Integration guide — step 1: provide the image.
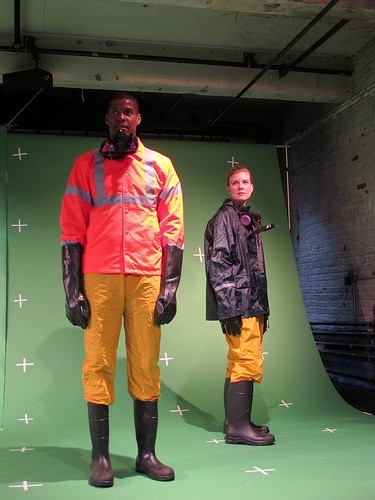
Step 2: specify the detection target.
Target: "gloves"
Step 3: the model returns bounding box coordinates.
[220,315,242,337]
[61,241,90,329]
[154,245,183,325]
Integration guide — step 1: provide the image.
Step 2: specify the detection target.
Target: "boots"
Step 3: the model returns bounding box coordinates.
[224,379,276,445]
[88,402,114,487]
[223,376,270,434]
[134,399,175,482]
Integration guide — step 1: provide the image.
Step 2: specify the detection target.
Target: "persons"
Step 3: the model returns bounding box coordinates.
[202,166,277,444]
[60,94,184,490]
